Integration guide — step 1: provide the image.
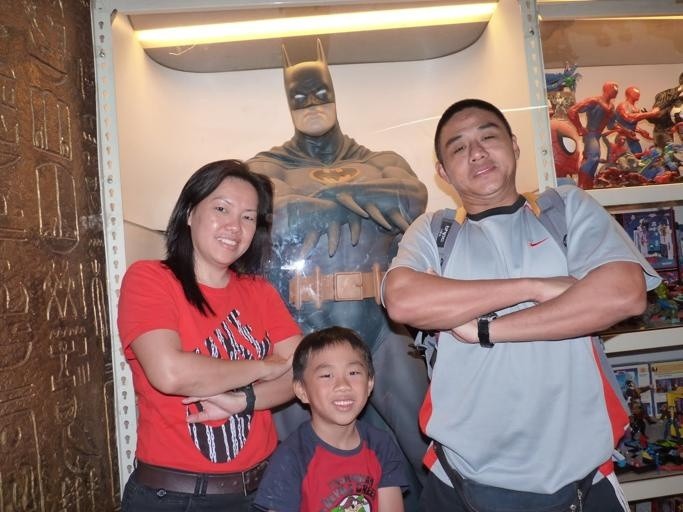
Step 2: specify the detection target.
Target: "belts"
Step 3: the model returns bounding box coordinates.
[135,460,269,497]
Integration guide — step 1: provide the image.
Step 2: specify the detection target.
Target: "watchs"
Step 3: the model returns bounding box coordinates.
[474,311,497,349]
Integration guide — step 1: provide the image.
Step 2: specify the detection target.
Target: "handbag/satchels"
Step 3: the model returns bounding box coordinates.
[453,473,590,511]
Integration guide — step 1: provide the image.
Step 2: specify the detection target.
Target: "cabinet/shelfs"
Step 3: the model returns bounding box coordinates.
[540,0,683,512]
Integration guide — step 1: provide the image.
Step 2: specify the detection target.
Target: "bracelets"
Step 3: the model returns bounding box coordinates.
[236,386,256,417]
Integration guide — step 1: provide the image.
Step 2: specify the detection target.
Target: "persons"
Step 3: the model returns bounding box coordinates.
[378,96,664,512]
[252,324,412,512]
[116,156,309,512]
